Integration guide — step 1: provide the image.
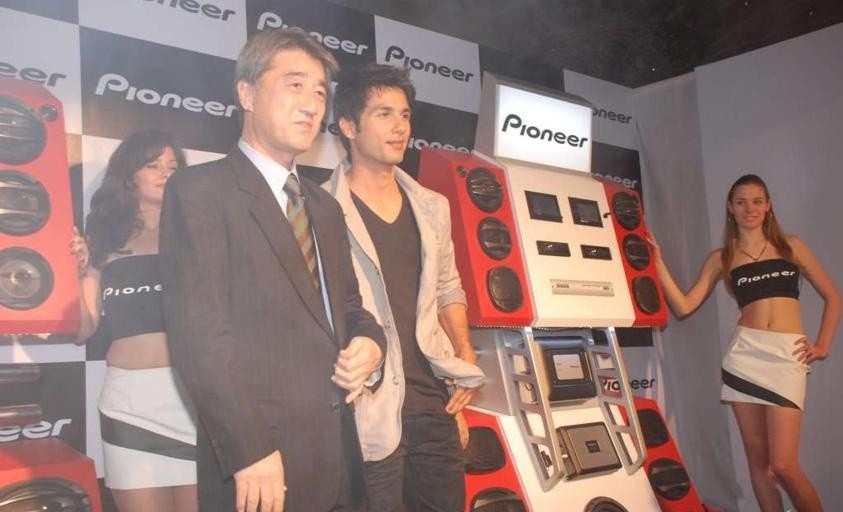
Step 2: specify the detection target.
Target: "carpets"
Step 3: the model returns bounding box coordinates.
[282,174,326,313]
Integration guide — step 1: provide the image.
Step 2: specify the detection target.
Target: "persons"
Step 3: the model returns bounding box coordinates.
[64,125,204,512]
[154,24,393,512]
[297,59,491,511]
[636,169,841,511]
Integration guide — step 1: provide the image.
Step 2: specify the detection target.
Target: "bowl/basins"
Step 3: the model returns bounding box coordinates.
[738,238,771,261]
